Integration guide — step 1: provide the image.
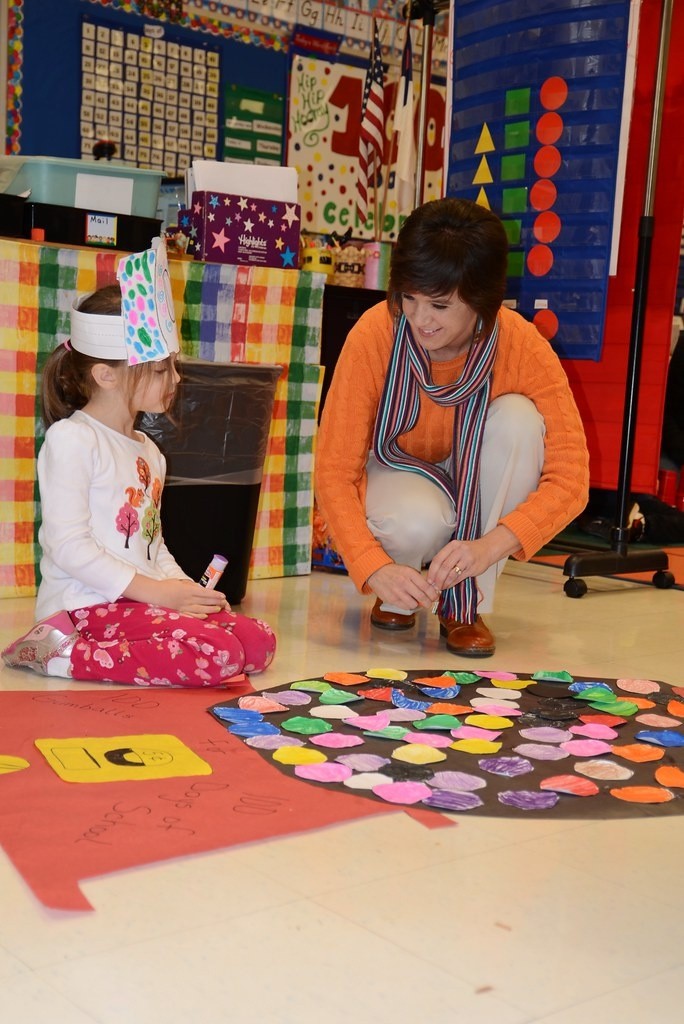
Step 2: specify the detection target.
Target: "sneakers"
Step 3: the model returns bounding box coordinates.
[0,610,81,678]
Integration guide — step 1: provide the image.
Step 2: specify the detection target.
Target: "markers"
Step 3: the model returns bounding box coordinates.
[199,555,228,590]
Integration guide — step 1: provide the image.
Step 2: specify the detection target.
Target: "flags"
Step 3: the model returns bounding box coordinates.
[359,23,417,219]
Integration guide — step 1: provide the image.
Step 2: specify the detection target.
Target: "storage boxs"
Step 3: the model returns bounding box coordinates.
[178,191,301,269]
[0,153,170,253]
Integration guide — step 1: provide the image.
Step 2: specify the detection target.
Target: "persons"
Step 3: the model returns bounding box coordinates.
[0,285,277,689]
[313,200,589,656]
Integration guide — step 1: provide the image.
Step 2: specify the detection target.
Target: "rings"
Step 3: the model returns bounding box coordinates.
[453,565,462,575]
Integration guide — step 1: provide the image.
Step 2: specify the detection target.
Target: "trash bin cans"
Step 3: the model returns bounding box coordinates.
[134,359,284,606]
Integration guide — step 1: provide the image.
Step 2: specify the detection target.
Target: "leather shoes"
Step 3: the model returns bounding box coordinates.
[371,595,416,630]
[438,612,495,656]
